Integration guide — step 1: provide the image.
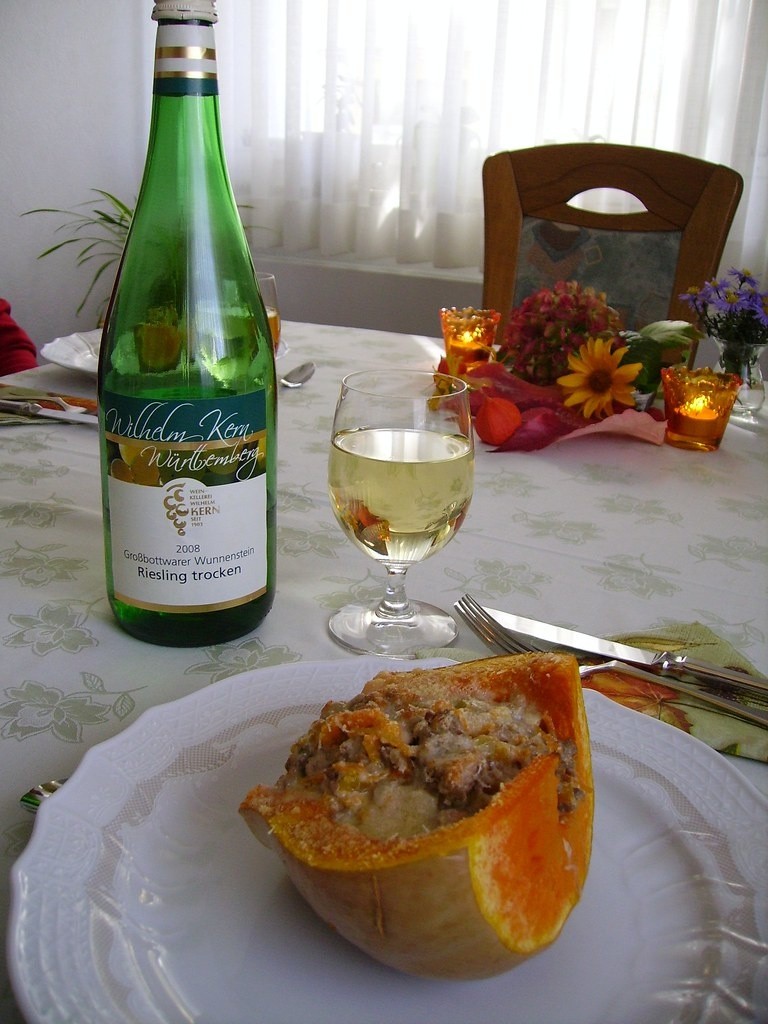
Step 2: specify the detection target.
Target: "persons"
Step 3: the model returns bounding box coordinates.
[1,297,40,378]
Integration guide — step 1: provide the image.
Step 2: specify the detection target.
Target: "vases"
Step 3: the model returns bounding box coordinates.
[712,336,766,418]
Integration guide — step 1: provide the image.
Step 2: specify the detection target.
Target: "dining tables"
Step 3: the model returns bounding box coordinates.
[1,318,767,1024]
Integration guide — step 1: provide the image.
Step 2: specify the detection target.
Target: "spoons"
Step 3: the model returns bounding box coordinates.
[281,360,317,387]
[0,393,97,415]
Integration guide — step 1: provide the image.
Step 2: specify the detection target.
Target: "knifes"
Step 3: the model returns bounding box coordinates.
[0,400,98,422]
[457,602,767,701]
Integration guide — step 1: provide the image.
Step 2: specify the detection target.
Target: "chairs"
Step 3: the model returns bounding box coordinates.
[481,142,745,371]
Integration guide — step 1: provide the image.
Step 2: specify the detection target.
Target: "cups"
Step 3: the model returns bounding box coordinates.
[661,366,742,451]
[253,270,281,358]
[441,307,502,382]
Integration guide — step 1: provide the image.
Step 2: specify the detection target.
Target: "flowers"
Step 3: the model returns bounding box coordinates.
[430,280,705,452]
[680,267,768,401]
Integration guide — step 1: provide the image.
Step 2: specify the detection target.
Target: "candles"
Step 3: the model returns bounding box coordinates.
[449,331,484,362]
[672,395,719,437]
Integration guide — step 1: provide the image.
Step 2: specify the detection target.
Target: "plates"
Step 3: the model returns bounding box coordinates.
[41,328,290,380]
[1,653,767,1024]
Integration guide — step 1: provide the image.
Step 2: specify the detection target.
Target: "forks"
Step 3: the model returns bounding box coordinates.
[452,594,768,728]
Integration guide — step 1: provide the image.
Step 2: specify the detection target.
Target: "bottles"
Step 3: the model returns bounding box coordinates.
[94,1,281,649]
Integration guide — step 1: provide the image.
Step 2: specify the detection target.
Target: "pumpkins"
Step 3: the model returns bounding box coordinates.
[244,630,596,981]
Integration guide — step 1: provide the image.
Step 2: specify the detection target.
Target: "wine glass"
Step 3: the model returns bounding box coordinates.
[328,370,475,658]
